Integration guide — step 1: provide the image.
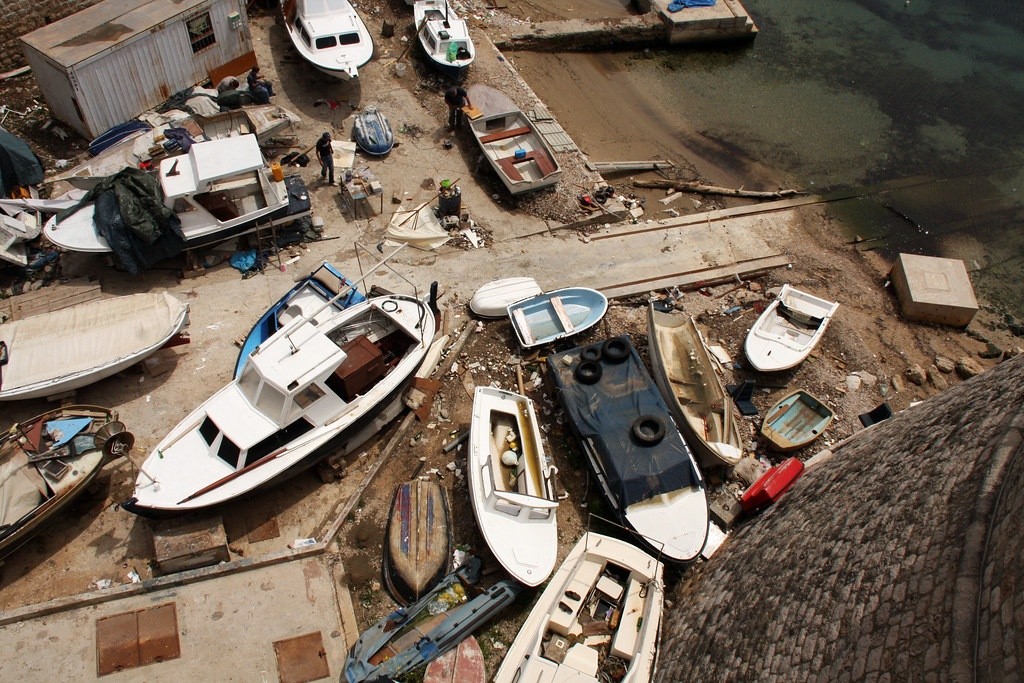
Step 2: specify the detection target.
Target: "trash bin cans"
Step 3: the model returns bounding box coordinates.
[438,185,461,217]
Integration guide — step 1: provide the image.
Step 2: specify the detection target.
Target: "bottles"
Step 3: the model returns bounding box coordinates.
[526,365,540,372]
[414,431,422,441]
[449,428,460,435]
[436,471,444,480]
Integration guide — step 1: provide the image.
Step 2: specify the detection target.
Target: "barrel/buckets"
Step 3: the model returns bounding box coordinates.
[438,188,461,219]
[271,161,284,182]
[396,63,406,77]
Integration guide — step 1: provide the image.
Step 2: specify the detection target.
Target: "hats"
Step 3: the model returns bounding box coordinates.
[323,133,330,140]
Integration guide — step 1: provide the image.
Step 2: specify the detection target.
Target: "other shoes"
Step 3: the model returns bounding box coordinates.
[268,92,277,96]
[323,173,327,180]
[329,182,338,186]
[447,125,454,132]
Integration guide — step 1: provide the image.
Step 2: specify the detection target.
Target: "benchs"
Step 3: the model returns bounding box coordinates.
[513,307,534,344]
[479,123,530,146]
[550,296,574,333]
[493,147,553,181]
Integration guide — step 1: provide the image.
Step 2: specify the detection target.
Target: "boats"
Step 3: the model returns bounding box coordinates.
[544,335,709,578]
[0,402,118,566]
[36,101,303,190]
[413,0,475,81]
[234,258,365,384]
[121,238,441,519]
[384,476,452,606]
[279,0,374,82]
[760,388,835,454]
[469,276,542,318]
[423,635,486,683]
[465,84,562,196]
[0,292,190,400]
[507,286,608,349]
[647,302,743,472]
[43,132,312,254]
[744,281,841,378]
[490,512,665,683]
[353,105,394,155]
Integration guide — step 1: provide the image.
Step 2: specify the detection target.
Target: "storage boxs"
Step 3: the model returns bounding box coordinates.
[152,515,231,574]
[889,253,979,329]
[329,335,385,398]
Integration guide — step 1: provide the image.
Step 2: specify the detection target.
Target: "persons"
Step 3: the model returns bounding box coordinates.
[445,86,473,133]
[247,68,276,104]
[217,77,240,95]
[316,132,339,186]
